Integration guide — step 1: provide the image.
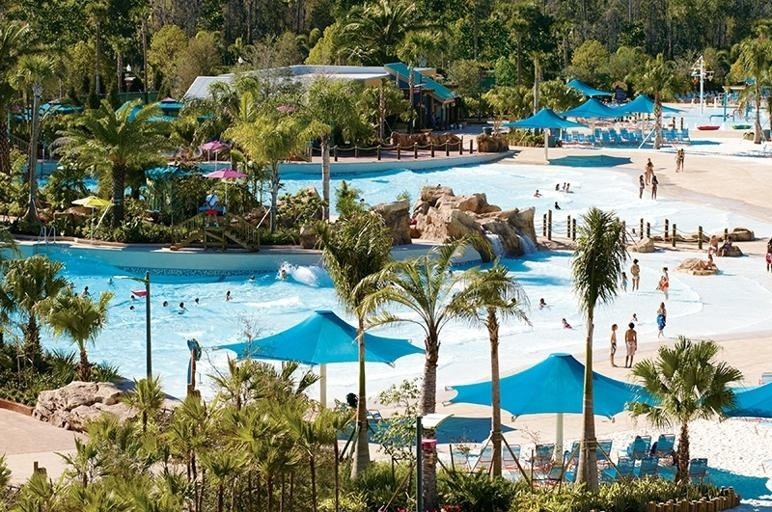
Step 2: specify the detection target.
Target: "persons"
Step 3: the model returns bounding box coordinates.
[562,318,571,328]
[431,112,437,126]
[533,182,572,209]
[638,158,658,199]
[690,235,732,270]
[631,259,640,292]
[205,189,220,229]
[657,267,669,291]
[620,272,628,292]
[73,267,287,315]
[632,313,637,321]
[539,299,546,309]
[657,302,667,338]
[766,238,772,272]
[674,149,684,173]
[610,323,637,366]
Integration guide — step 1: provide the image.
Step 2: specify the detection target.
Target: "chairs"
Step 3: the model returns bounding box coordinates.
[554,127,692,147]
[453,434,708,485]
[333,398,384,423]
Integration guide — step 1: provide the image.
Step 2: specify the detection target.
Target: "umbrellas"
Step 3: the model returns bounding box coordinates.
[70,196,114,240]
[556,98,637,146]
[441,353,663,463]
[215,309,426,409]
[565,79,613,97]
[198,139,233,171]
[202,167,249,206]
[618,94,689,143]
[502,107,589,161]
[698,379,772,419]
